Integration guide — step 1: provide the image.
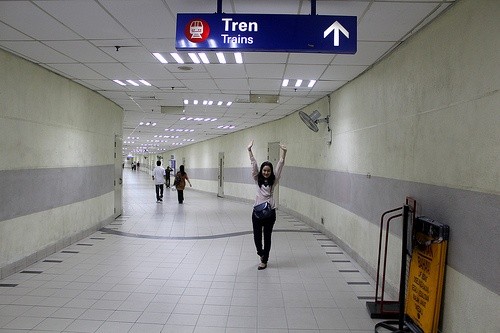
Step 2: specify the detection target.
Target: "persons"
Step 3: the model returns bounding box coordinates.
[247,137,288,271]
[131,160,140,172]
[151,160,167,204]
[164,165,173,188]
[173,165,193,205]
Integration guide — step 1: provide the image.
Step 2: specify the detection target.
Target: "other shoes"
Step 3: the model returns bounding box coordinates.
[257,250,267,270]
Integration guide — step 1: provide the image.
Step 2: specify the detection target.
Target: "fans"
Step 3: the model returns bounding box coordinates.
[156,155,162,161]
[298,109,330,134]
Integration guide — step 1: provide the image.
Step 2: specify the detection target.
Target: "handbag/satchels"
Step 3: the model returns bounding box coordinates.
[254,201,272,220]
